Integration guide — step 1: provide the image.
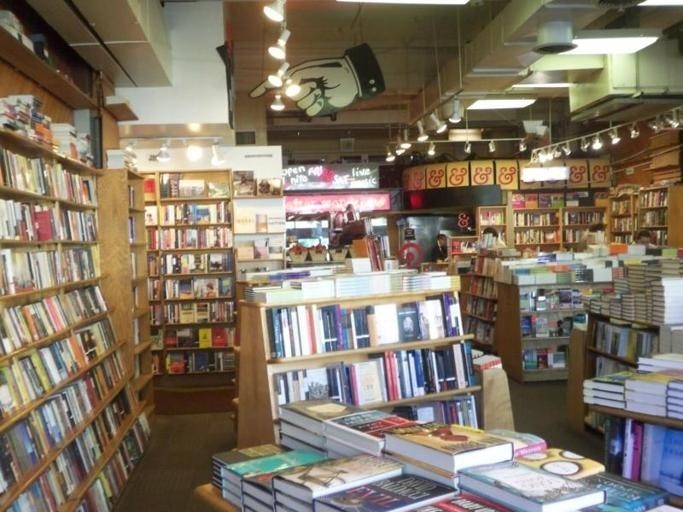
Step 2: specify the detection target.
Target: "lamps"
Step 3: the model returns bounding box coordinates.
[530,106,683,167]
[559,26,664,57]
[467,95,538,110]
[263,1,301,113]
[385,4,465,162]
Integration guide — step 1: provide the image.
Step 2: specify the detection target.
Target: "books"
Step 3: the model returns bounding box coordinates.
[143,174,236,375]
[212,257,670,512]
[0,94,151,512]
[584,353,683,498]
[453,189,683,377]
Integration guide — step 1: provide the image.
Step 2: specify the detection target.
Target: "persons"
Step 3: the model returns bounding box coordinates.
[432,234,448,263]
